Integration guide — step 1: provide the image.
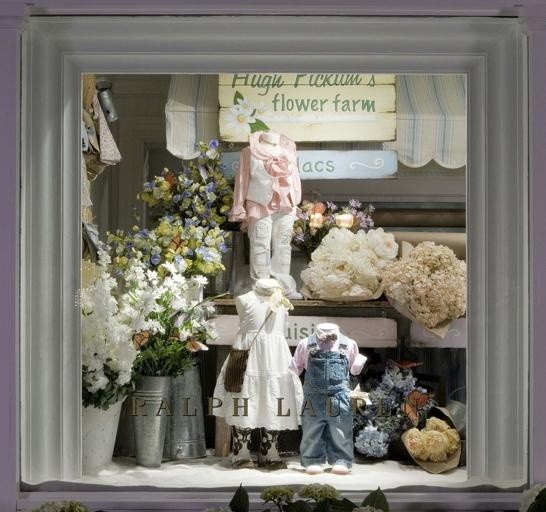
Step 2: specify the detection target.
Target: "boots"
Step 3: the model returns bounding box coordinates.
[231,426,254,469]
[257,427,288,470]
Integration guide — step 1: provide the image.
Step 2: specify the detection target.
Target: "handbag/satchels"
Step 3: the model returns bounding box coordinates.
[225,348,249,393]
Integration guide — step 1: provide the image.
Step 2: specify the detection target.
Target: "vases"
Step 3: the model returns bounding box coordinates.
[165,364,206,459]
[131,375,170,466]
[82,398,128,473]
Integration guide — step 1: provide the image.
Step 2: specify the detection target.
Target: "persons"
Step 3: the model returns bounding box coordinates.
[212,279,304,469]
[228,128,303,302]
[286,322,369,475]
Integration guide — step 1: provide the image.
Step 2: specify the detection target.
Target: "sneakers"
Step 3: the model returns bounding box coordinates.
[330,465,348,475]
[284,291,304,300]
[306,464,323,474]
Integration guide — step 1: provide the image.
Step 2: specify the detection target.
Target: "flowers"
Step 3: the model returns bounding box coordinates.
[71,138,238,412]
[399,417,463,475]
[221,482,389,512]
[224,91,272,131]
[350,363,438,460]
[289,196,467,340]
